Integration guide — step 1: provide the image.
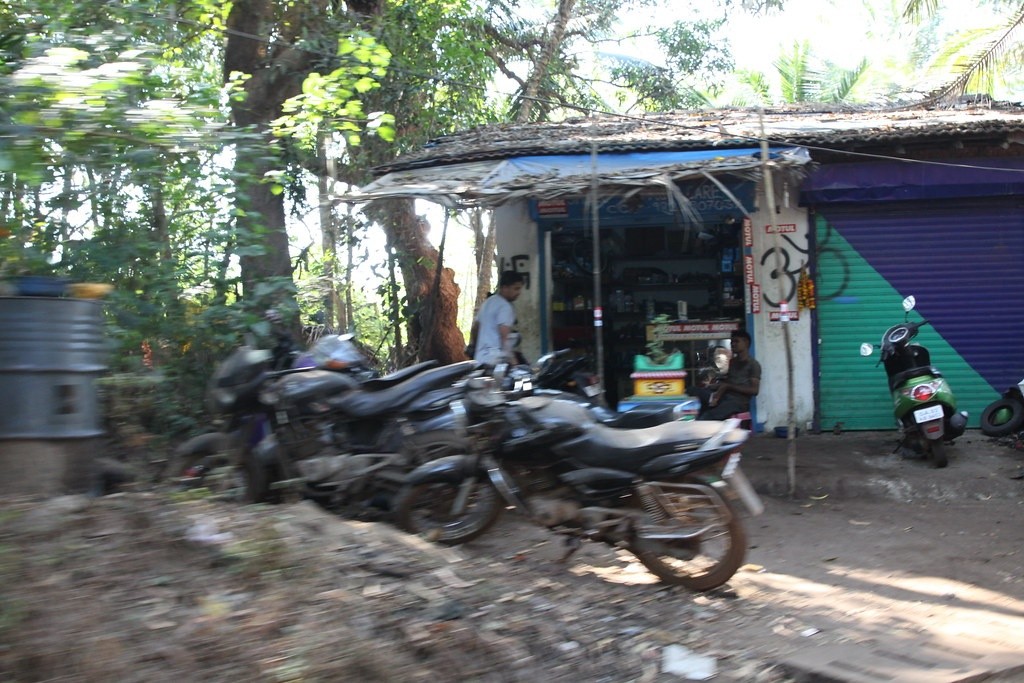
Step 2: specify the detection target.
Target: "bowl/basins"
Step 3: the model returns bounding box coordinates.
[0,272,69,296]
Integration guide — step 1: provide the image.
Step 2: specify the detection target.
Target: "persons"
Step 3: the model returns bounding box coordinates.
[472,272,528,367]
[687,330,761,421]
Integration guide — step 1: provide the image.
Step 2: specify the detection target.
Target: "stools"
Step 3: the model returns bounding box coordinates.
[730,411,754,432]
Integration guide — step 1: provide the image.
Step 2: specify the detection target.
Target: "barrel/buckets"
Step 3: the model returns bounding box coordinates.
[0,296,106,499]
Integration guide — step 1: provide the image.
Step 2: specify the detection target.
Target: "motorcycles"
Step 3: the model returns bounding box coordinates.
[158,308,765,591]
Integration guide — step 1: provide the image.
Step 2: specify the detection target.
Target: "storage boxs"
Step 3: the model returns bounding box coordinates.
[620,372,700,419]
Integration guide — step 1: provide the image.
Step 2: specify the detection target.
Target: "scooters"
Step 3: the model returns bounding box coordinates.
[859,295,970,470]
